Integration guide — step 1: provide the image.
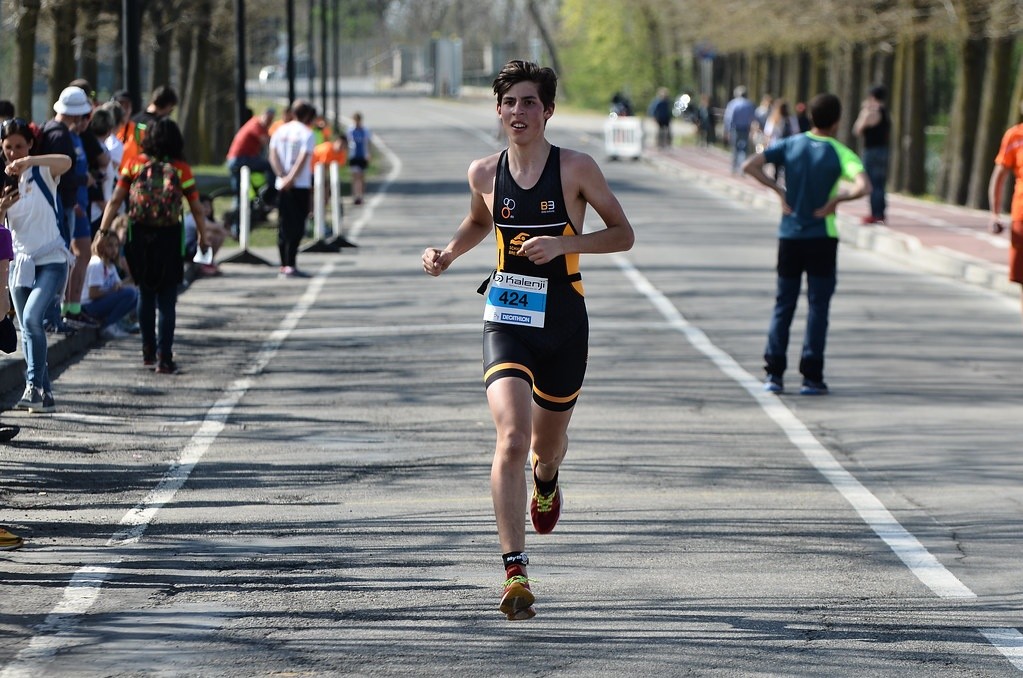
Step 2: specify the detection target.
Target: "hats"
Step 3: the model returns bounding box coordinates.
[53,87,92,115]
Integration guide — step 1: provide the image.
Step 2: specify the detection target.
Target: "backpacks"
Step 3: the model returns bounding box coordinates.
[129,153,181,225]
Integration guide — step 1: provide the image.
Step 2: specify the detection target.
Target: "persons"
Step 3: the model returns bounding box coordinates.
[68,79,92,98]
[269,102,317,279]
[266,105,294,137]
[1,116,72,413]
[421,59,635,621]
[102,101,126,179]
[38,87,93,335]
[721,85,754,178]
[110,214,139,333]
[226,105,276,240]
[346,112,372,204]
[988,94,1023,285]
[608,85,715,163]
[763,100,800,184]
[91,108,115,238]
[92,120,213,374]
[851,88,890,224]
[111,90,145,174]
[308,135,348,220]
[743,93,872,398]
[184,193,225,277]
[134,85,185,160]
[80,231,140,339]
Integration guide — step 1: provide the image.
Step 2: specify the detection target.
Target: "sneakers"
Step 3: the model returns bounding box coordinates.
[157,362,177,373]
[500,565,537,620]
[280,266,312,278]
[531,452,562,534]
[18,388,43,407]
[144,359,159,369]
[108,323,131,338]
[28,392,56,412]
[62,311,100,329]
[801,385,829,394]
[765,382,783,392]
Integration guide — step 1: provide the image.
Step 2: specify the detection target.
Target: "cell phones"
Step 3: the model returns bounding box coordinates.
[6,174,19,198]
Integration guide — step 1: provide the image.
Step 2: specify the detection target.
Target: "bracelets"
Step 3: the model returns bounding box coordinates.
[98,227,108,238]
